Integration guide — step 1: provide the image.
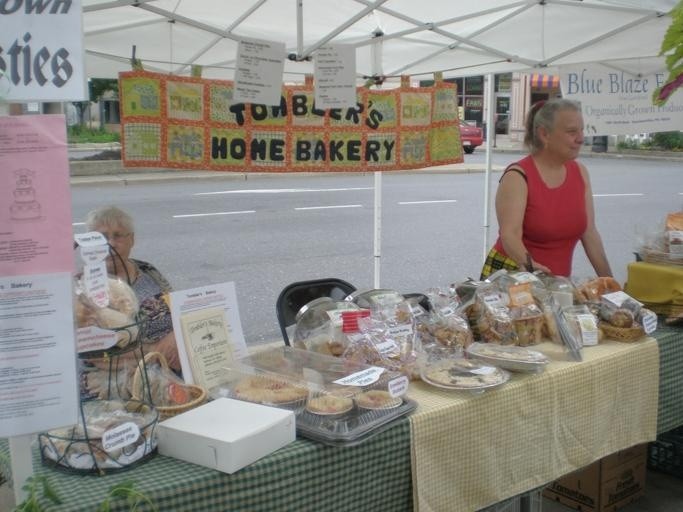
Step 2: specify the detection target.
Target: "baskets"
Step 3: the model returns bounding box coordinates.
[95,350,209,423]
[597,317,646,343]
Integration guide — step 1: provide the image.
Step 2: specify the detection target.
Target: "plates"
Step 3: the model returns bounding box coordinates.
[465,343,546,374]
[421,365,511,394]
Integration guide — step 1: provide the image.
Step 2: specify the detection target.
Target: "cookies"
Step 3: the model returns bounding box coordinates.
[308,275,640,380]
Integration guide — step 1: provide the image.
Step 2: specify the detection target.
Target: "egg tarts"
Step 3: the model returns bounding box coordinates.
[358,389,400,415]
[235,374,280,400]
[307,395,355,420]
[265,381,310,406]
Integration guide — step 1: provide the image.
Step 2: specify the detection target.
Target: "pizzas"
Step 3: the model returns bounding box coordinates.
[426,342,542,387]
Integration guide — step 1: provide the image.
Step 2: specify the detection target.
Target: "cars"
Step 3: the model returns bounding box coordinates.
[459,117,484,153]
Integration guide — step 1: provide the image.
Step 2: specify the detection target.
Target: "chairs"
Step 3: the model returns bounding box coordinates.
[274,279,361,347]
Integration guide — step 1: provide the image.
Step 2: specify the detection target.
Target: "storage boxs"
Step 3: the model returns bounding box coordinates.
[538,441,647,512]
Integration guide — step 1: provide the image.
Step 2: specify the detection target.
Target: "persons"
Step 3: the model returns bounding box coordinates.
[71,204,181,401]
[482,95,612,279]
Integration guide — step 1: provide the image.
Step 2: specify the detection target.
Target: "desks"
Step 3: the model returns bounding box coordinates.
[623,253,683,323]
[409,323,658,512]
[30,376,409,511]
[648,321,682,436]
